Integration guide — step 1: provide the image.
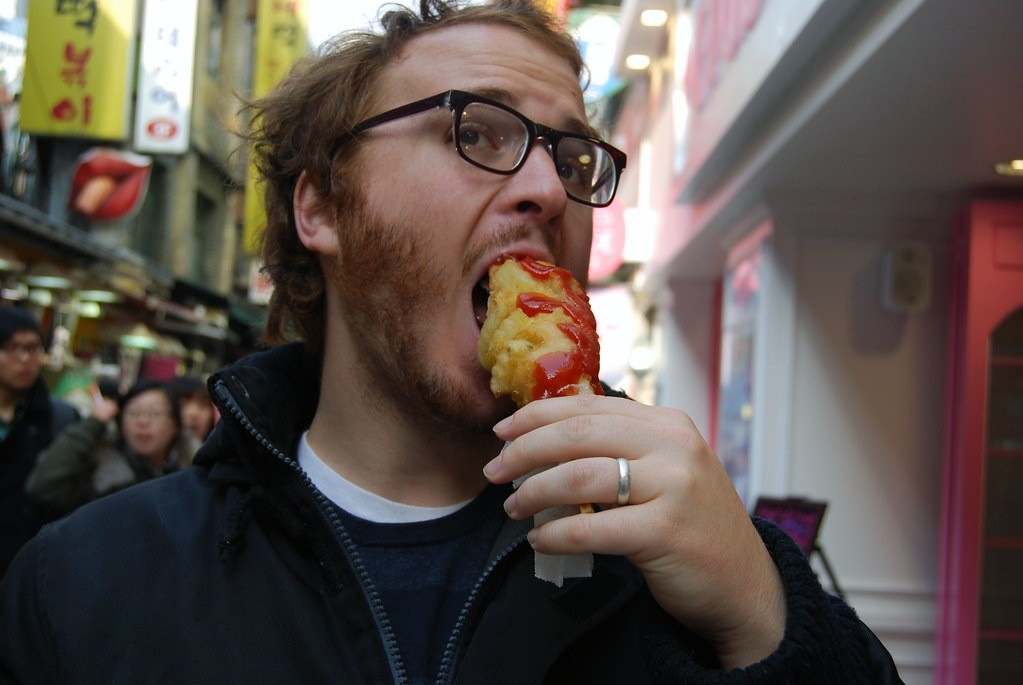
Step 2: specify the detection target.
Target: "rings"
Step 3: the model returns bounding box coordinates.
[618,457,631,505]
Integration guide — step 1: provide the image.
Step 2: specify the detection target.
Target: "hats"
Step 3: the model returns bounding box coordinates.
[0,305,41,355]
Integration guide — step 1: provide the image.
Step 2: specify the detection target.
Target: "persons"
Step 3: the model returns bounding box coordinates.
[0,0,907,683]
[1,302,222,549]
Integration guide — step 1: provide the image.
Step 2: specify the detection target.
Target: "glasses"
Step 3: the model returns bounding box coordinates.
[322,90,629,207]
[0,345,45,362]
